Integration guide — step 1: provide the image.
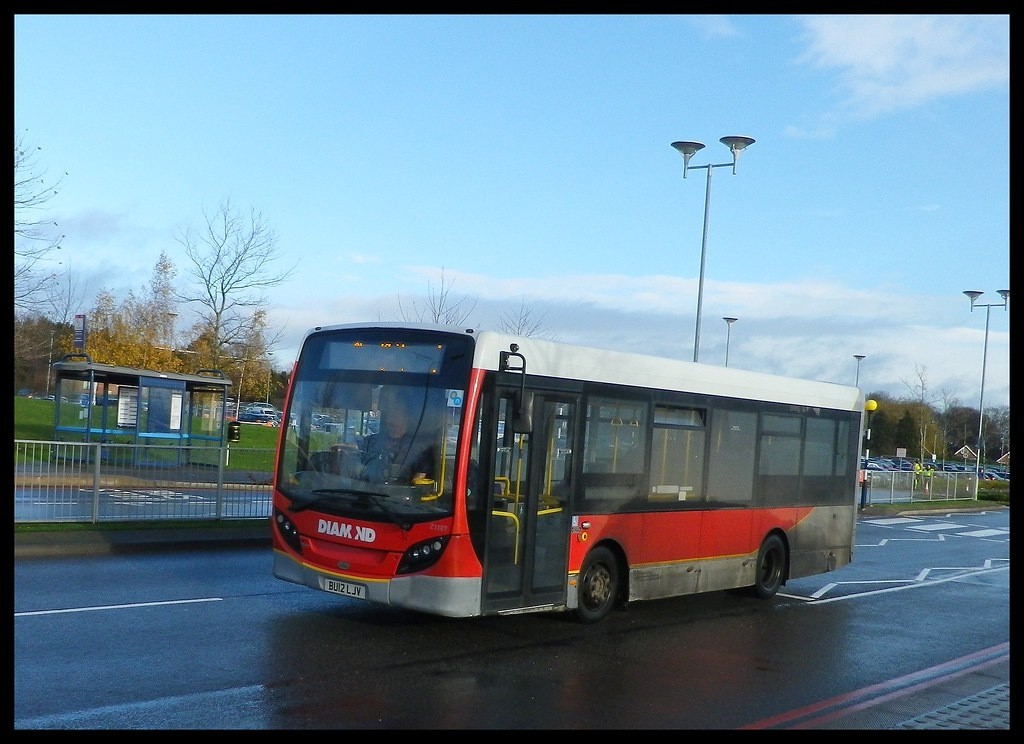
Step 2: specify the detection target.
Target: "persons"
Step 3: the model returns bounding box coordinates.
[336,407,432,484]
[913,461,923,490]
[922,464,934,484]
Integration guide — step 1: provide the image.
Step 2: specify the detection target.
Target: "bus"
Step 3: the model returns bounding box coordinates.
[270,321,866,619]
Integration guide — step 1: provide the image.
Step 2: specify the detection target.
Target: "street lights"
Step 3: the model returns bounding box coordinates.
[861,400,878,511]
[853,355,866,387]
[168,313,179,360]
[671,135,757,363]
[962,288,1009,501]
[723,317,738,367]
[46,330,56,396]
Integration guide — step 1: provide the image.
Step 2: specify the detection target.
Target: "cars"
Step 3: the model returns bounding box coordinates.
[225,398,380,436]
[31,394,69,403]
[861,458,1009,481]
[17,389,46,399]
[97,395,118,407]
